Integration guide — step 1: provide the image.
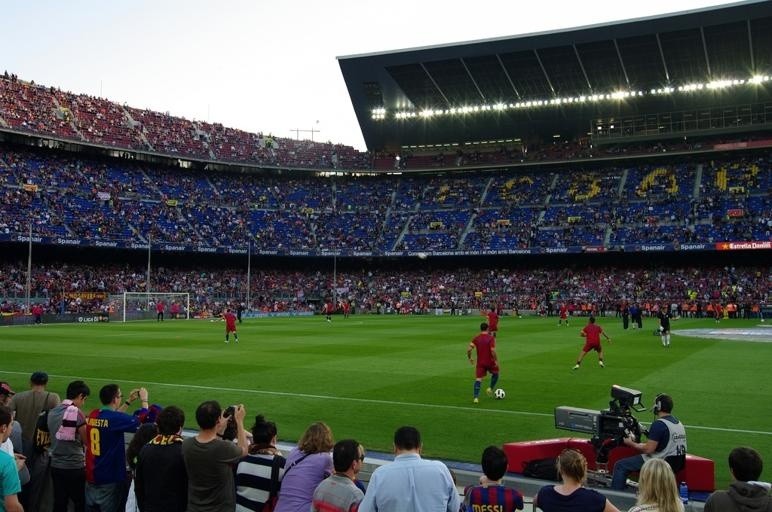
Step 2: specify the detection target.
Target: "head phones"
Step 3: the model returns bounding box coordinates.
[654,393,666,410]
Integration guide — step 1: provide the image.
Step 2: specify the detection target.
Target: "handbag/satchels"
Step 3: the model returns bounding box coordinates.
[33,410,51,453]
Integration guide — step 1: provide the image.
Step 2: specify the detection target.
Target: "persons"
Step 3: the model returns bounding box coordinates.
[656,302,681,347]
[572,315,612,371]
[1,370,770,511]
[1,69,770,329]
[465,322,499,404]
[214,309,241,343]
[486,304,501,344]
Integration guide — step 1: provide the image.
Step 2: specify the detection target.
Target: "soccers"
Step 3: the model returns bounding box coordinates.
[493,388,504,398]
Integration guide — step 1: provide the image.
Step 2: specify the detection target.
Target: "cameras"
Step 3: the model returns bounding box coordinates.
[224,406,235,417]
[137,391,140,396]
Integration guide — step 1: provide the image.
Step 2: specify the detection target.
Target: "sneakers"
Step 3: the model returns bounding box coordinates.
[599,361,604,368]
[487,387,493,399]
[474,397,478,403]
[572,364,579,370]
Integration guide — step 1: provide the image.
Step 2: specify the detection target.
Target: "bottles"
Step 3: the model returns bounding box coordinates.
[678,481,688,505]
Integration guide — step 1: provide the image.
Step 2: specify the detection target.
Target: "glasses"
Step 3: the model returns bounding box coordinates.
[359,454,364,461]
